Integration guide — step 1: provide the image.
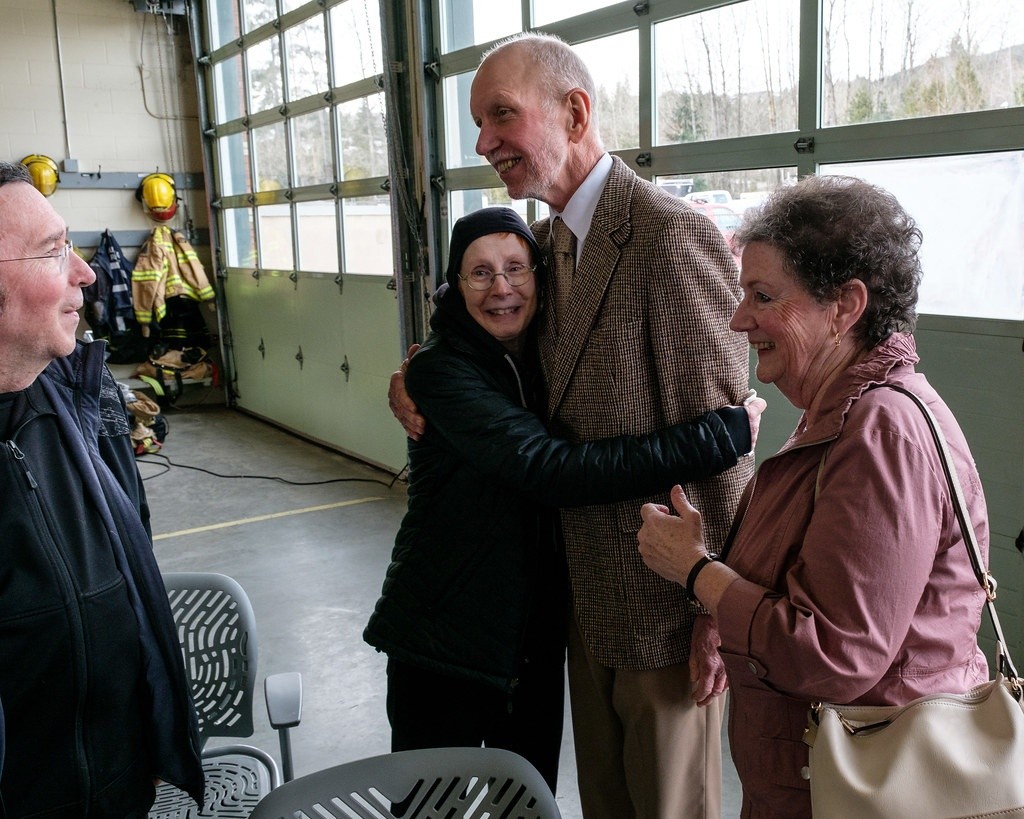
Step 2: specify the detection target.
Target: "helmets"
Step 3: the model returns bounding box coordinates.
[135,174,179,222]
[18,155,60,198]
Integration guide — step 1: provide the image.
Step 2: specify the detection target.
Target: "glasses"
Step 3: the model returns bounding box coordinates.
[0,240,73,272]
[457,261,537,291]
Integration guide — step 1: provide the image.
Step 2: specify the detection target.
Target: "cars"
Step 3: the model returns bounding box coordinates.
[681,190,771,270]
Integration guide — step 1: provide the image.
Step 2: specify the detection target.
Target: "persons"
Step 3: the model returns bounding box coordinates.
[363,33,766,819]
[637,172,991,819]
[1,161,205,819]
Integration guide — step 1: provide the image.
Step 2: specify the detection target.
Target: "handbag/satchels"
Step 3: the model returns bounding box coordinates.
[800,383,1024,819]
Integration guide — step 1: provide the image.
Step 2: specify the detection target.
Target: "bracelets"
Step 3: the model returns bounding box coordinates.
[684,551,721,612]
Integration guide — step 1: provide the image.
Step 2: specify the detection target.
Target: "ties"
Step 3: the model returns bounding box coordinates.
[551,217,577,331]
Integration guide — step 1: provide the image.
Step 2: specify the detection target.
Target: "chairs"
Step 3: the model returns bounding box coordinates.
[145,571,563,819]
